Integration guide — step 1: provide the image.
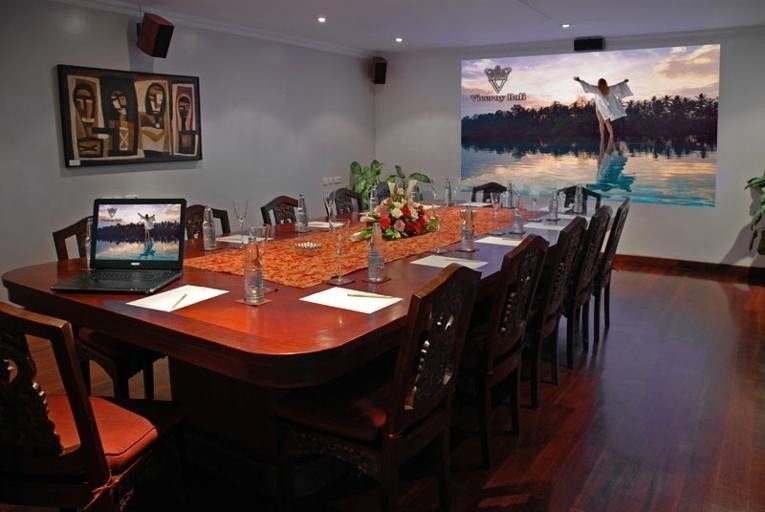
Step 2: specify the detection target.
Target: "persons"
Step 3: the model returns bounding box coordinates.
[574,76,633,165]
[137,212,156,242]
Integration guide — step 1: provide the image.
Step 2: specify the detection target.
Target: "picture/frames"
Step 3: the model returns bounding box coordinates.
[56,64,203,169]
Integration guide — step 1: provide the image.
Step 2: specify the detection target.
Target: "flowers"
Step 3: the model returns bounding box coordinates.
[359,188,434,240]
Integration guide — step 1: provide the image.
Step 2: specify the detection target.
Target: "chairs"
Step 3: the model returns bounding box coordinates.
[261,194,300,226]
[2,302,160,512]
[51,216,167,400]
[469,182,521,208]
[280,262,482,512]
[325,189,363,217]
[530,183,633,409]
[184,205,231,242]
[376,182,423,203]
[451,234,549,435]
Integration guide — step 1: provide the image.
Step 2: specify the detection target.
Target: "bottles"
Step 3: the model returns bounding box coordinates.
[366,222,386,283]
[369,188,378,216]
[202,204,216,251]
[84,216,93,270]
[294,192,308,233]
[409,177,583,254]
[241,236,264,304]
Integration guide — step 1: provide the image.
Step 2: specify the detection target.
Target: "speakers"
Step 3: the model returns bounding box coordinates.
[135,11,174,58]
[371,56,386,85]
[573,38,604,50]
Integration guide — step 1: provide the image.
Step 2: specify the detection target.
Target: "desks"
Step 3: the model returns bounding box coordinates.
[1,205,548,389]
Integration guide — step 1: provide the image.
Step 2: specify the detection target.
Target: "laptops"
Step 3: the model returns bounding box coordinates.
[48,197,187,295]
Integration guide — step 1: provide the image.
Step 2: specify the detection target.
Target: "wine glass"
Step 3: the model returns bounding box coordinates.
[325,217,354,286]
[231,199,249,250]
[247,224,277,295]
[322,187,337,233]
